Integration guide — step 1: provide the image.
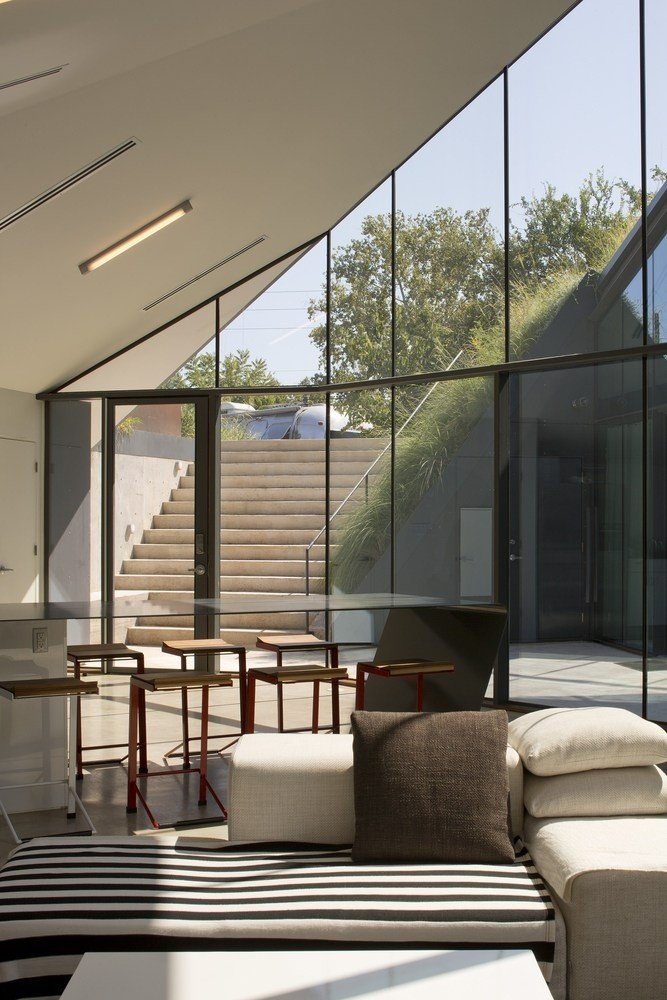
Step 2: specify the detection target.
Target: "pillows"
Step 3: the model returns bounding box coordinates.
[350,710,516,863]
[507,707,667,776]
[523,763,667,818]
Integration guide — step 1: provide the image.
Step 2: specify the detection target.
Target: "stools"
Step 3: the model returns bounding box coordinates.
[354,658,456,712]
[162,638,247,770]
[126,671,233,829]
[0,677,99,845]
[66,643,148,780]
[247,664,349,735]
[256,634,340,735]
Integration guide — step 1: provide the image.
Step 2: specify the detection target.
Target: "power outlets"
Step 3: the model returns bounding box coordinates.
[32,628,49,654]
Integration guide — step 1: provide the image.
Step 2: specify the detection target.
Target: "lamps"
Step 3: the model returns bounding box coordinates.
[77,199,194,275]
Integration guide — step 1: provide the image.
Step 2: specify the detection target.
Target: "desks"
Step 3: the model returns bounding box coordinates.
[0,593,509,711]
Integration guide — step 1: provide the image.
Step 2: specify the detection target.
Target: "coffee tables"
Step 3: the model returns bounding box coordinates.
[58,949,555,999]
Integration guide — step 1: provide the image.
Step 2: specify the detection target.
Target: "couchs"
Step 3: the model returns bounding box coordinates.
[0,733,667,999]
